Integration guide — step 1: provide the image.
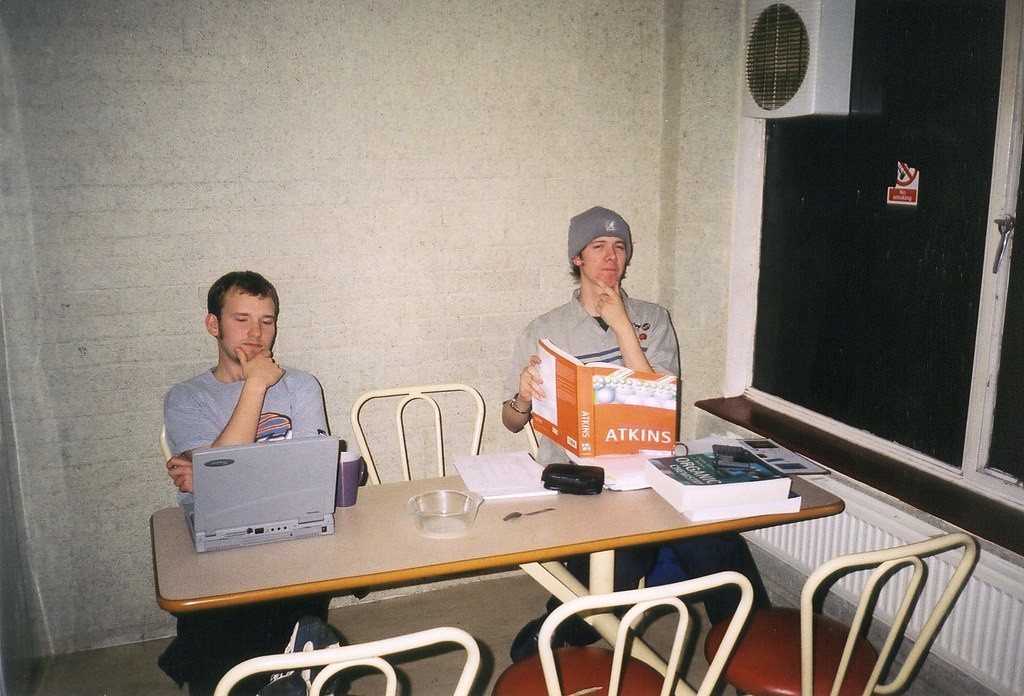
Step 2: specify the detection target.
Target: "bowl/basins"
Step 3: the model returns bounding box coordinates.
[408,489,484,538]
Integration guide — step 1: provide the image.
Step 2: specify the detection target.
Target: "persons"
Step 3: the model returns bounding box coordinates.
[500,206,773,662]
[163,269,354,696]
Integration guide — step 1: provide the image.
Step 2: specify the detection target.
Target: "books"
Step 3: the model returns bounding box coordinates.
[644,451,802,524]
[689,437,831,479]
[526,338,680,460]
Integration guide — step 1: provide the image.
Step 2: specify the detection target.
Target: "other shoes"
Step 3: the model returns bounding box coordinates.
[256,617,341,696]
[511,613,560,664]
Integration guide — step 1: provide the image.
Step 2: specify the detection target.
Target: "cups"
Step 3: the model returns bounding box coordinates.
[335,452,366,508]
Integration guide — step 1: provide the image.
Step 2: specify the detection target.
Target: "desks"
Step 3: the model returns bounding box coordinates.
[149,475,844,696]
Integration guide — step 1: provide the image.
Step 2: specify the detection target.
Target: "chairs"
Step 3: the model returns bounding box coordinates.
[705,532,977,696]
[492,571,754,696]
[213,627,480,696]
[351,384,484,486]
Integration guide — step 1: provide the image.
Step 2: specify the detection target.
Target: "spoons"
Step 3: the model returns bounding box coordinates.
[503,507,555,521]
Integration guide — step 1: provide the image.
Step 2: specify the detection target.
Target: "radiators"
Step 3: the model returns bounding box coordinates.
[742,497,1024,696]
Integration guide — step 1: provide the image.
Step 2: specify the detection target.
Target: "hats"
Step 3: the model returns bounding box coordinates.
[568,206,633,266]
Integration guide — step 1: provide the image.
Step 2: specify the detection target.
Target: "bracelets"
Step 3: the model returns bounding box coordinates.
[510,393,533,414]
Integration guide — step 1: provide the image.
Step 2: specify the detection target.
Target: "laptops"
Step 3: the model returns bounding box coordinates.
[183,435,339,552]
